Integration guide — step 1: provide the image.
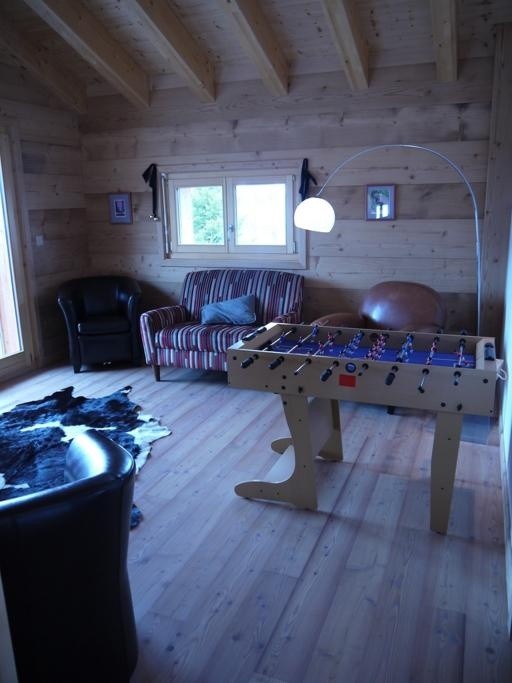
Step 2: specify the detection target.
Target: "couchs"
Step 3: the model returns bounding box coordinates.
[139,269,305,381]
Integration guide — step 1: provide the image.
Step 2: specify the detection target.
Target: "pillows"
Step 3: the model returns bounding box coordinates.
[200,294,257,325]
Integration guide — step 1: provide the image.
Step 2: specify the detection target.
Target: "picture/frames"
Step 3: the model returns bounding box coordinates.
[365,184,396,220]
[108,192,133,224]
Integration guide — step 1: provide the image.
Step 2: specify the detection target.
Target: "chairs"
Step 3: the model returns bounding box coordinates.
[0,430,139,683]
[309,281,447,413]
[57,274,144,373]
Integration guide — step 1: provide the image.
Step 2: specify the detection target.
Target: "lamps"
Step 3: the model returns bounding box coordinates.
[293,143,479,337]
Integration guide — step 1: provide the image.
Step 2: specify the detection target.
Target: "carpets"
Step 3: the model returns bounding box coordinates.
[0,384,172,531]
